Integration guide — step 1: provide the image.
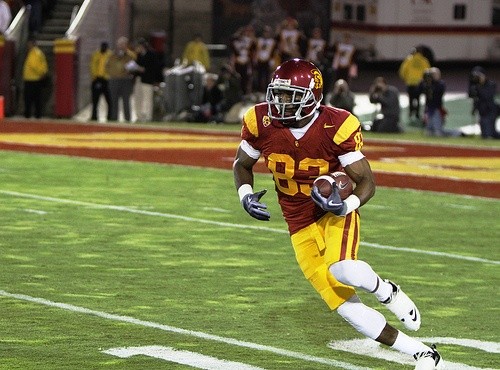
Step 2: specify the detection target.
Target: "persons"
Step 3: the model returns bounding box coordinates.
[231,15,356,115]
[182,34,211,69]
[90,32,174,123]
[468,65,500,138]
[398,47,461,136]
[23,36,48,119]
[202,75,224,124]
[369,76,404,133]
[232,57,446,370]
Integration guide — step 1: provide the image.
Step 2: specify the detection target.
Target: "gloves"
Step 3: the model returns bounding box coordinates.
[309,181,346,218]
[242,189,270,223]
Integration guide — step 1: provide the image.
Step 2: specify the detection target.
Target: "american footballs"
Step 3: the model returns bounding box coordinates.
[311,172,354,202]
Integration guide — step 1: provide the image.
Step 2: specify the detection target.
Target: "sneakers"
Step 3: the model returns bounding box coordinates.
[379,278,421,331]
[412,344,441,370]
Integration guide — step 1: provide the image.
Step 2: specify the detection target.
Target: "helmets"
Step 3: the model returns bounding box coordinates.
[264,58,323,121]
